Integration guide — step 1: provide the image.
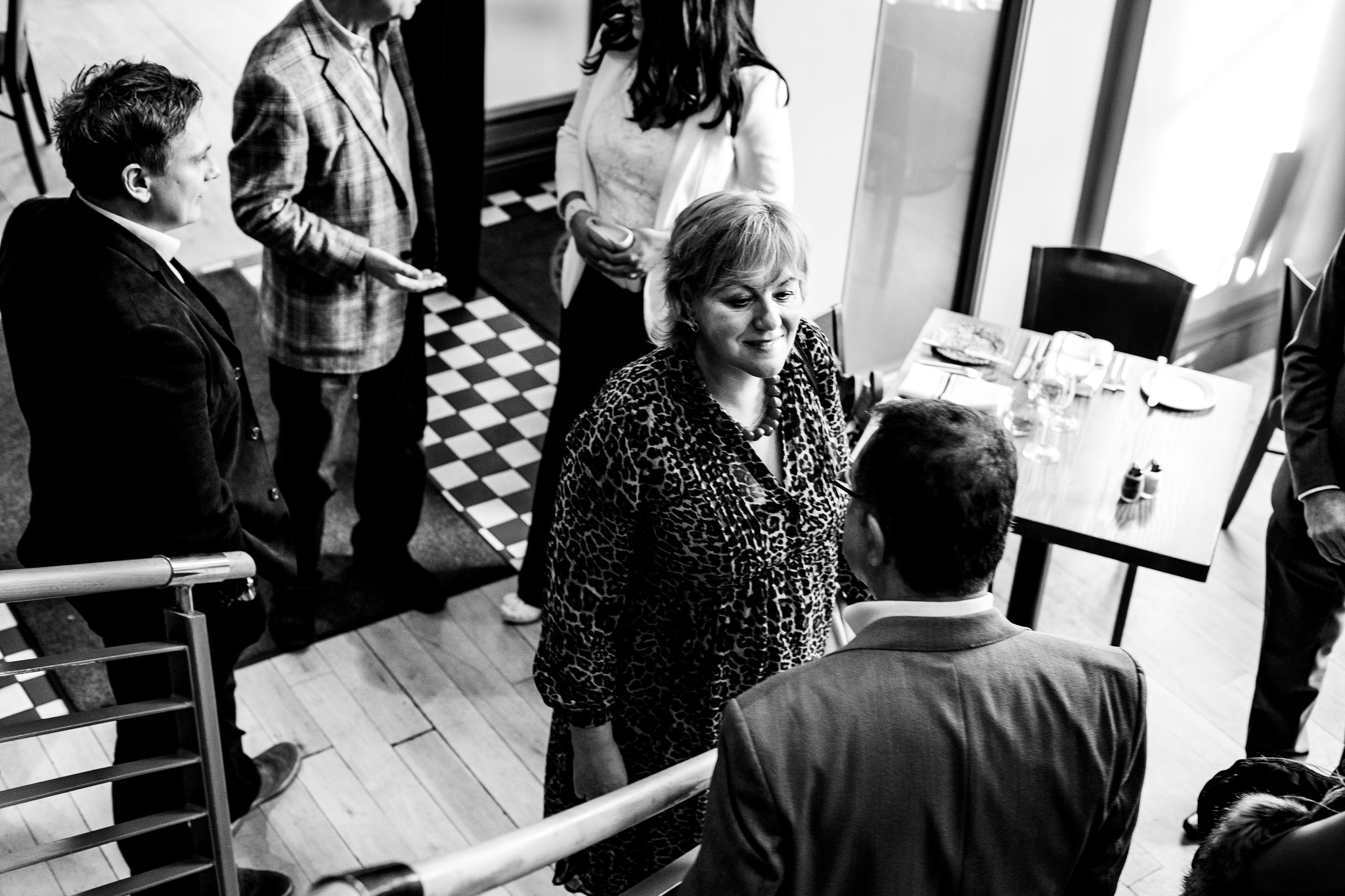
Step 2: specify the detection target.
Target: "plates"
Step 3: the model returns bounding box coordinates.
[936,322,1003,365]
[1141,369,1214,411]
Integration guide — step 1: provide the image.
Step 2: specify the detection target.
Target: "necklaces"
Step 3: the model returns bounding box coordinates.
[686,333,782,440]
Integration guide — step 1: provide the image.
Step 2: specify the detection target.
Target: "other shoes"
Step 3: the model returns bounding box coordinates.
[270,580,319,651]
[366,559,448,616]
[498,591,544,624]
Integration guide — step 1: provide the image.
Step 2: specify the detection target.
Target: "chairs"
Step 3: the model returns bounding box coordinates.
[1019,247,1195,364]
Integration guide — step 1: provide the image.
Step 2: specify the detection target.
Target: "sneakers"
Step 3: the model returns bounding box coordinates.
[227,738,304,839]
[209,868,294,895]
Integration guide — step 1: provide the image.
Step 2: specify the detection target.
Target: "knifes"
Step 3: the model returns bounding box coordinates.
[1012,333,1041,380]
[1146,355,1168,406]
[920,336,1013,366]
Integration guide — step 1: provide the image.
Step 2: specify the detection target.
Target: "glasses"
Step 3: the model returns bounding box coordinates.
[824,465,900,517]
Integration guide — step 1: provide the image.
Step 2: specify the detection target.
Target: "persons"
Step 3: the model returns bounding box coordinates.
[533,188,879,896]
[0,59,305,896]
[1181,746,1345,895]
[681,398,1148,896]
[1180,223,1345,836]
[502,0,787,626]
[227,0,446,652]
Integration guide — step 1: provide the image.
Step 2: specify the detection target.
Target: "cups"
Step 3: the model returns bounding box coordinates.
[585,215,635,250]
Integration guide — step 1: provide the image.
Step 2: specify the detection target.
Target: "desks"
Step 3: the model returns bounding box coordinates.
[850,307,1251,632]
